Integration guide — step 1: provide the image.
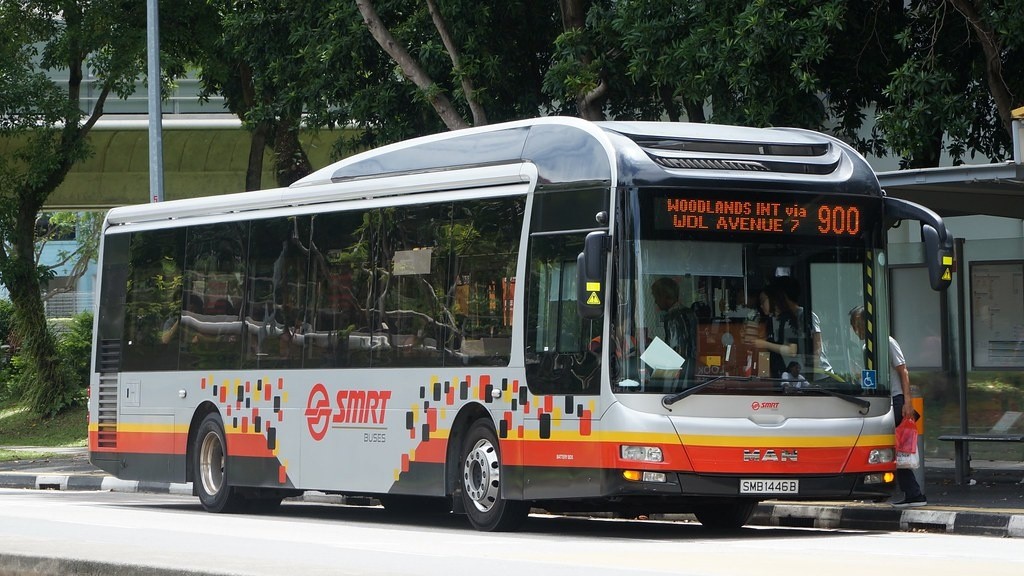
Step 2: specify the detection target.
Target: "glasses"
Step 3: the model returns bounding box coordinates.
[757,296,768,306]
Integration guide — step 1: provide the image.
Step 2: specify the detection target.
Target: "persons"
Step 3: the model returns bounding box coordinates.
[651,278,698,392]
[588,314,652,359]
[162,295,305,355]
[849,304,927,507]
[750,274,840,383]
[781,361,810,393]
[691,285,798,378]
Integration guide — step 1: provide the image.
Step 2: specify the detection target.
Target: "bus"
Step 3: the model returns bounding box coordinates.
[86,113,960,534]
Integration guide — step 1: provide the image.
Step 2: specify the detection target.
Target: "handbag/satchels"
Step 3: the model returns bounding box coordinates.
[895,428,921,470]
[895,416,918,453]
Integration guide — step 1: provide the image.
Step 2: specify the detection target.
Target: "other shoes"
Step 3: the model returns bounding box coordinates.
[891,494,927,507]
